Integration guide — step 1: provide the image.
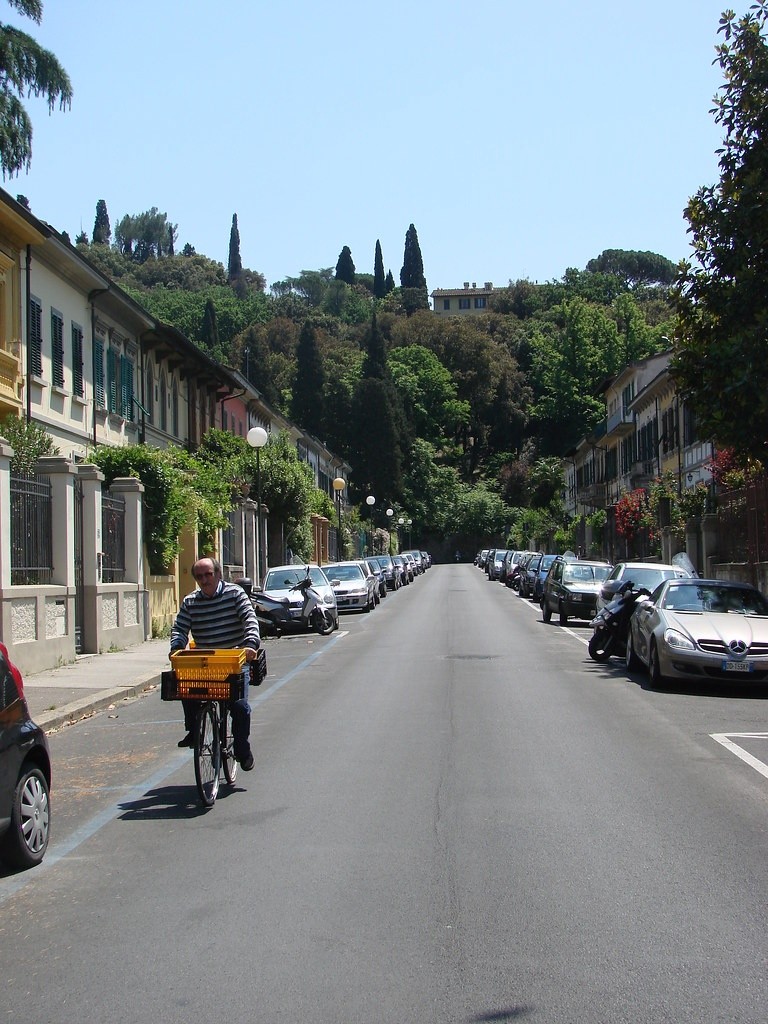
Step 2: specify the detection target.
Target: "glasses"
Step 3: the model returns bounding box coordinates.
[194,572,218,581]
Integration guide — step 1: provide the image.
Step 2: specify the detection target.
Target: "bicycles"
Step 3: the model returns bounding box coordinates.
[169,649,247,806]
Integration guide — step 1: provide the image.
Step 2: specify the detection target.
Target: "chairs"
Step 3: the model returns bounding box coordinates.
[348,570,359,578]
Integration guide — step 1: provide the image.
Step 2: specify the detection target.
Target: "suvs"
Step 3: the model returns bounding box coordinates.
[539,556,616,626]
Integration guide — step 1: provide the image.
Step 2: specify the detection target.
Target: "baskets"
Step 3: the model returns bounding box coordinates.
[161,672,244,702]
[248,648,268,686]
[171,649,246,699]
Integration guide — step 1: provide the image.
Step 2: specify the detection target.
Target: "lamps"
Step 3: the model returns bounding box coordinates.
[688,470,700,482]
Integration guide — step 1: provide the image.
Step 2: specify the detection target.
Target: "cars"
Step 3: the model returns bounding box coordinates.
[474,548,515,581]
[363,554,417,591]
[0,643,51,864]
[595,562,702,610]
[319,560,386,613]
[253,565,340,629]
[626,578,768,684]
[500,550,579,602]
[401,550,431,573]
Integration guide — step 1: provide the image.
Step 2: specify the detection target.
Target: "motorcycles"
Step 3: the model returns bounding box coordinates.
[234,566,335,639]
[588,580,652,660]
[455,554,461,562]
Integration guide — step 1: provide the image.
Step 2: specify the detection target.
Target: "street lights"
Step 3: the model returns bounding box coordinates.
[408,519,412,549]
[247,426,268,587]
[386,509,393,555]
[399,518,404,551]
[366,496,375,556]
[333,478,346,561]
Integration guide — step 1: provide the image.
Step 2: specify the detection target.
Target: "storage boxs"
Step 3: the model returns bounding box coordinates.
[170,650,246,698]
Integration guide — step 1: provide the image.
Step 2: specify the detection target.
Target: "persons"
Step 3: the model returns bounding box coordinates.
[169,557,260,771]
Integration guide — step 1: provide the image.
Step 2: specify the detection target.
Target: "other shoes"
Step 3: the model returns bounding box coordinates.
[178,731,203,748]
[236,745,254,771]
